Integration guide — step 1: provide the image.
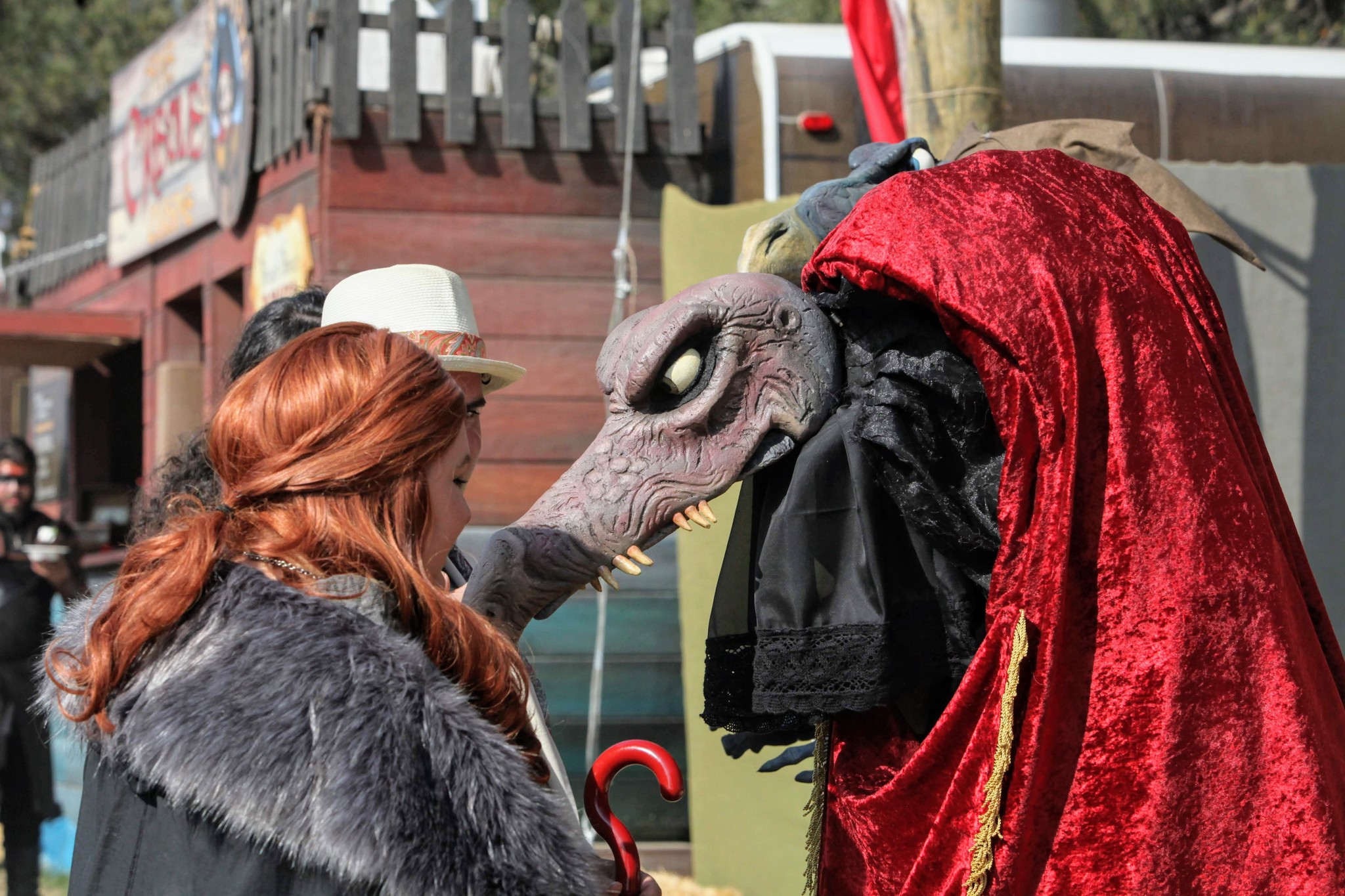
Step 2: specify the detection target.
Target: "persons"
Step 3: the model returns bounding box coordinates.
[0,432,91,896]
[40,321,663,896]
[320,263,529,589]
[133,293,329,528]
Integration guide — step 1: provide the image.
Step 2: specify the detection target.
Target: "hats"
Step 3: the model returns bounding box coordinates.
[323,264,527,387]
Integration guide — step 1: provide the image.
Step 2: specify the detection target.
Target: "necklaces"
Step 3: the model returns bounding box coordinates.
[244,552,316,579]
